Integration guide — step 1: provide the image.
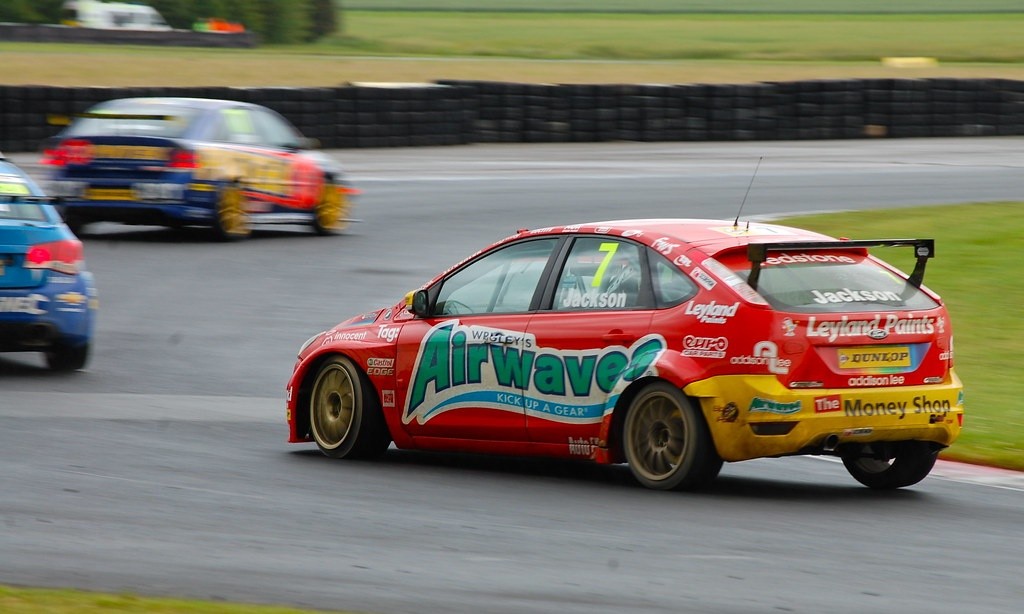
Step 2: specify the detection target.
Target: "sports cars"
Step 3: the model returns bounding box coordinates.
[1,153,99,373]
[35,94,350,244]
[286,215,964,492]
